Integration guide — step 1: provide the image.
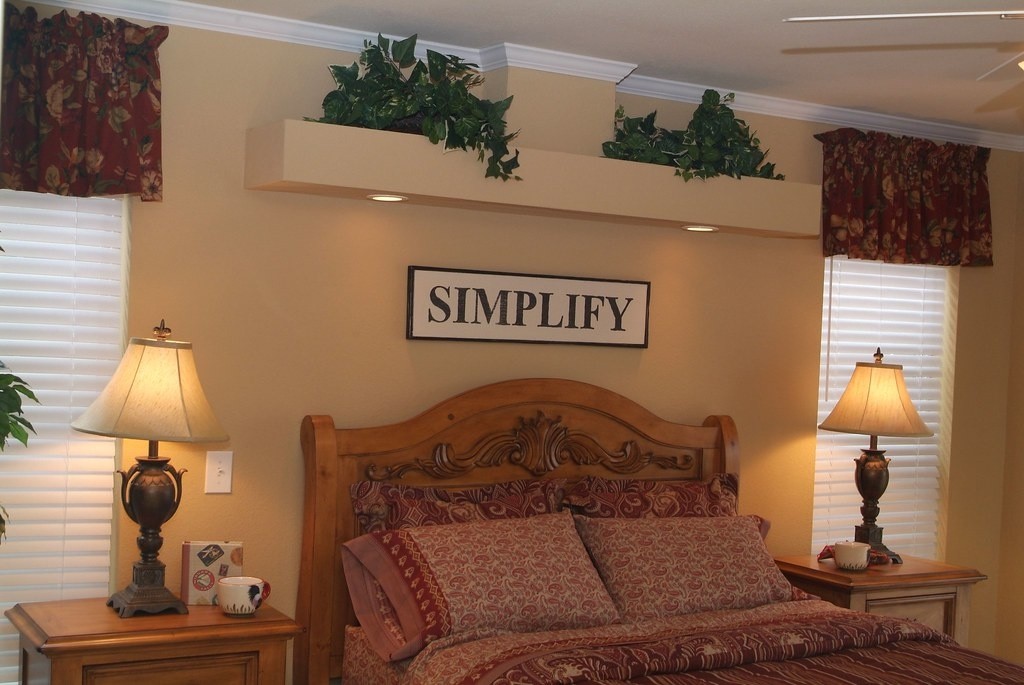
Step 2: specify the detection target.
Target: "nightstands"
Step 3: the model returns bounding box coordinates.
[3,598,307,685]
[773,555,989,649]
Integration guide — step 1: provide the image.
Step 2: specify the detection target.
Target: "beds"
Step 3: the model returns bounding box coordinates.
[292,377,1024,684]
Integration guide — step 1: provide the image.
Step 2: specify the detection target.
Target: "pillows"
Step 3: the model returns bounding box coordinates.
[351,480,564,537]
[573,514,813,624]
[547,471,738,520]
[340,508,619,664]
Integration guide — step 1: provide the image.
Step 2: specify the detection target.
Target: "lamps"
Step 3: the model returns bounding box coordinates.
[817,348,934,564]
[70,319,229,618]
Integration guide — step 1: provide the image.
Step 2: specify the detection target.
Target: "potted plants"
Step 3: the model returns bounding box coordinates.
[304,32,524,184]
[601,88,787,184]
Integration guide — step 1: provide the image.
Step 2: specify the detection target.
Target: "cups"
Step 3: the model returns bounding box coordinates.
[218,575,271,618]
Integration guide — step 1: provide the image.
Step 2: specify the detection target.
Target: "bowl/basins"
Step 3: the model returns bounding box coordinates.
[834,541,871,570]
